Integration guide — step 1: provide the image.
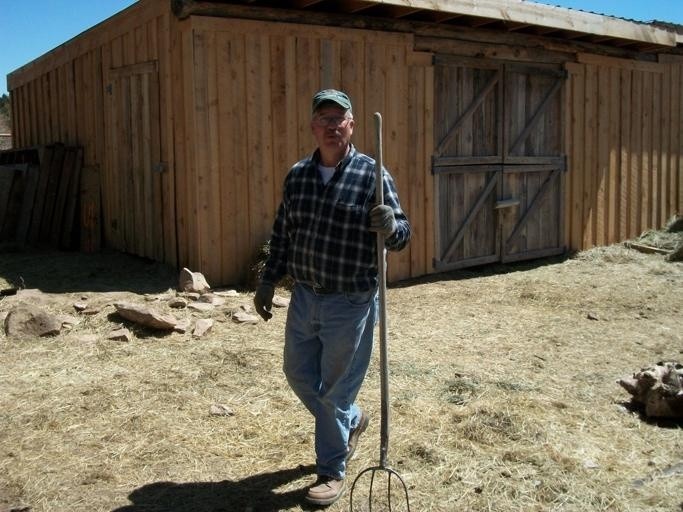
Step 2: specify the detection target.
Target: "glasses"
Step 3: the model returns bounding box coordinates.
[312,116,352,127]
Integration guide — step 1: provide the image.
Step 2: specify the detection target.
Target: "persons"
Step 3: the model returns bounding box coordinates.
[253,90,411,506]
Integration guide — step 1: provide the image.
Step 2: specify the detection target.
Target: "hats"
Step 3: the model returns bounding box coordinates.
[311,87,353,113]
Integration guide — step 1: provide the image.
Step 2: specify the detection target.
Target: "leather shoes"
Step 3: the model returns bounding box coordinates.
[304,472,349,508]
[344,411,369,465]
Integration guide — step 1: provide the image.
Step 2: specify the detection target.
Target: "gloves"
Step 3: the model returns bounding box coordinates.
[367,203,399,240]
[252,284,276,322]
